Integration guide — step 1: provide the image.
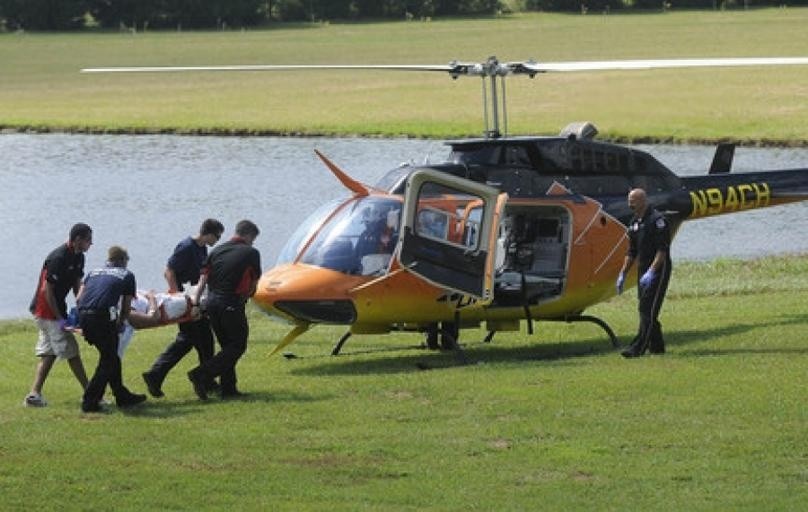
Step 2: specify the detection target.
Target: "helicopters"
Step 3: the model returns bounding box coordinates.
[79,55,806,371]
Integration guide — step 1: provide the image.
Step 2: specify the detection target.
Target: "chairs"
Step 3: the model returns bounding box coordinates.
[504,238,565,289]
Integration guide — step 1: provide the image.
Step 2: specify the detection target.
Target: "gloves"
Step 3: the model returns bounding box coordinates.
[616,272,623,294]
[639,272,653,289]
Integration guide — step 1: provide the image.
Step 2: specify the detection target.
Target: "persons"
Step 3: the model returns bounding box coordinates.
[117,284,213,331]
[140,218,225,399]
[355,208,392,257]
[75,246,148,414]
[615,186,675,359]
[426,322,460,350]
[25,221,111,409]
[188,219,264,402]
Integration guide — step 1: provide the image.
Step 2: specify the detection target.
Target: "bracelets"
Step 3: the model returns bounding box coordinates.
[190,302,201,307]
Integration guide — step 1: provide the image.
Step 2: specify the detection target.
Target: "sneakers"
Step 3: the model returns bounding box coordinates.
[81,393,146,411]
[188,371,245,398]
[24,394,47,407]
[621,346,645,356]
[143,370,165,396]
